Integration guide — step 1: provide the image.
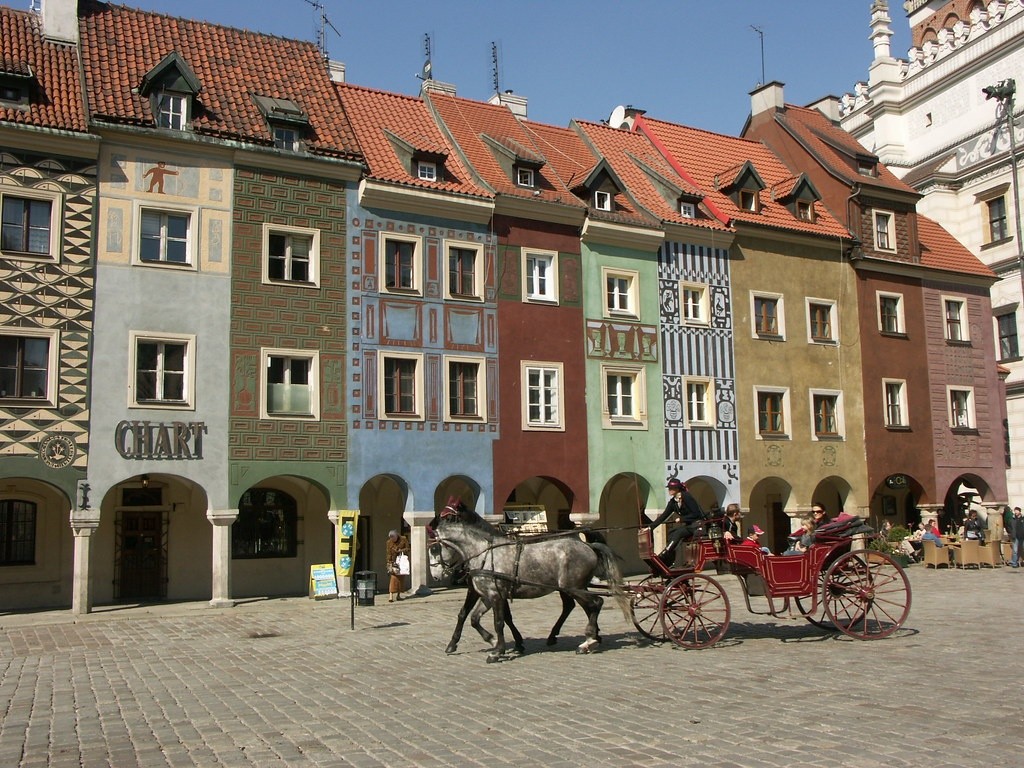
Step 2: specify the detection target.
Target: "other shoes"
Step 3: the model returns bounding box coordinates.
[397,598,404,601]
[389,600,393,602]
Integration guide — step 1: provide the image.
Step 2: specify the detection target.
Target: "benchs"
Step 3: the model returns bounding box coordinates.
[787,514,875,571]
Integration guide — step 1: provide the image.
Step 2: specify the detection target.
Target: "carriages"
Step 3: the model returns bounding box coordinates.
[424,494,913,656]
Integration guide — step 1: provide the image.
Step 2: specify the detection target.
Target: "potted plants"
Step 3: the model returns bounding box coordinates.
[886,525,911,568]
[866,539,892,564]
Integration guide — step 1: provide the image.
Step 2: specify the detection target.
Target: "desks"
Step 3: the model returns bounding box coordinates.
[944,542,960,546]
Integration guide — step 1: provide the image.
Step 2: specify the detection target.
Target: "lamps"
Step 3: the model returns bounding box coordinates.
[141,474,150,491]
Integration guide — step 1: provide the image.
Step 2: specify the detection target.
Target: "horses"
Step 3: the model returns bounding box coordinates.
[426,494,635,663]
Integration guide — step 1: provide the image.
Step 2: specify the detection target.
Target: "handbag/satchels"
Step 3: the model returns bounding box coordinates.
[386,564,399,575]
[396,552,410,576]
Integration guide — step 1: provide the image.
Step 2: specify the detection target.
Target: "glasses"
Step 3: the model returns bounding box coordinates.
[812,510,824,514]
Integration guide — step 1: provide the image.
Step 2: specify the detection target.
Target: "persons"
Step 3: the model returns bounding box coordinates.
[881,518,954,566]
[955,510,986,546]
[782,503,833,557]
[723,503,774,555]
[386,530,410,603]
[136,535,155,603]
[1008,507,1024,568]
[644,478,711,569]
[938,510,957,534]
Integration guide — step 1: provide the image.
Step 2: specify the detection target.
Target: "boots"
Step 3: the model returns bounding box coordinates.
[659,541,678,559]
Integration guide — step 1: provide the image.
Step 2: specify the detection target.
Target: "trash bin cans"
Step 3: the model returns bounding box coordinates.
[355,570,378,606]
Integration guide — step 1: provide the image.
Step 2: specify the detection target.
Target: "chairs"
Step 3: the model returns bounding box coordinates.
[683,513,726,566]
[978,540,1003,569]
[953,539,981,570]
[1003,542,1023,567]
[921,539,950,570]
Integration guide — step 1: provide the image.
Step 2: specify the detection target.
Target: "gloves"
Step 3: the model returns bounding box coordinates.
[724,531,734,540]
[641,526,651,532]
[675,518,680,523]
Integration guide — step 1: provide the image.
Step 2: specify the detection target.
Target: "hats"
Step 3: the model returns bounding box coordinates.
[666,479,688,493]
[748,524,764,535]
